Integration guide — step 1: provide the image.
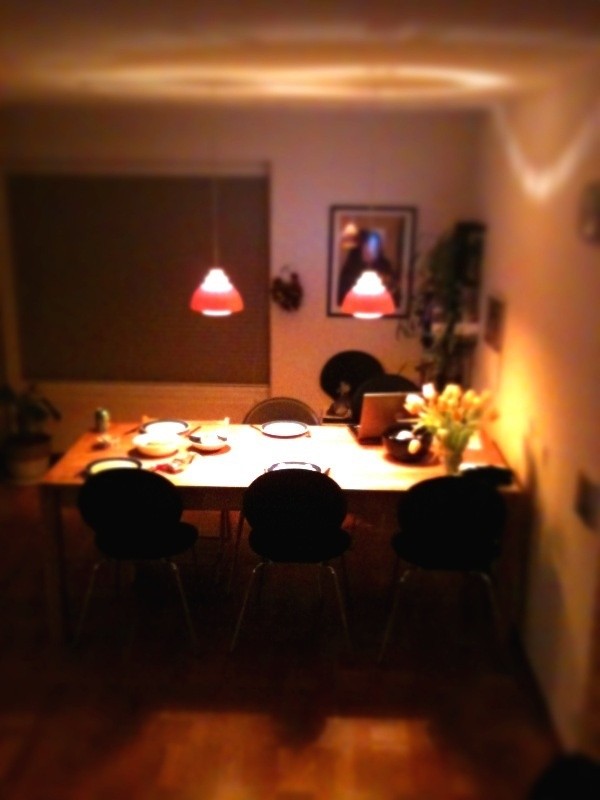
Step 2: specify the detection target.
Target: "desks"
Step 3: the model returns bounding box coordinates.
[32,412,520,664]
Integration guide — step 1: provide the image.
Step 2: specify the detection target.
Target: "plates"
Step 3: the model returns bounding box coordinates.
[262,421,309,436]
[87,457,142,475]
[267,462,321,472]
[139,419,189,434]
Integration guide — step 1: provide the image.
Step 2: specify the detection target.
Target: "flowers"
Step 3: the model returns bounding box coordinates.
[393,380,503,468]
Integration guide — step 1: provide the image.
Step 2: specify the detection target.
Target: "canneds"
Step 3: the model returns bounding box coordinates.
[95,407,109,431]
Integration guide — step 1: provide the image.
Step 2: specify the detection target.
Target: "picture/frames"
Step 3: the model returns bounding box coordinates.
[324,200,415,321]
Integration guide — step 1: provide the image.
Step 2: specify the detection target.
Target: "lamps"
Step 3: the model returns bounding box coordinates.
[188,171,246,319]
[342,269,401,323]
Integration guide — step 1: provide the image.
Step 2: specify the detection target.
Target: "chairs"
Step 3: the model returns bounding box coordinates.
[68,469,208,658]
[225,467,362,661]
[242,397,319,424]
[371,474,524,666]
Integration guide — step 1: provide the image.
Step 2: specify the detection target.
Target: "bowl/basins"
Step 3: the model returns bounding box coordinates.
[192,436,228,450]
[133,434,181,456]
[383,426,433,462]
